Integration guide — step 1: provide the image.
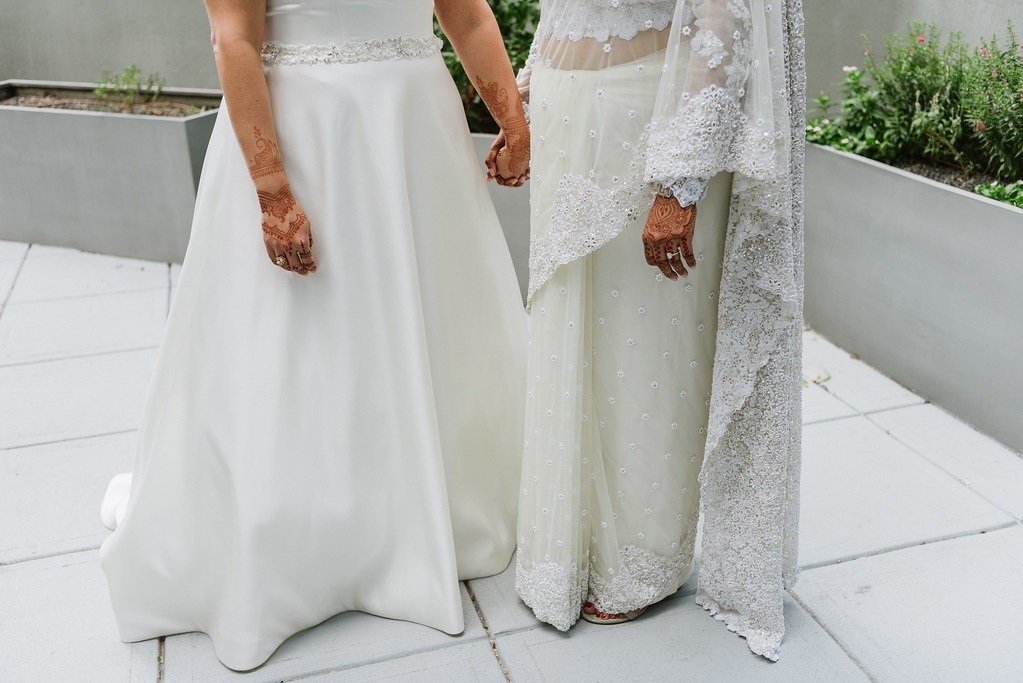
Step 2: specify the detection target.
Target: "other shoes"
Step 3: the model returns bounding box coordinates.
[581,603,645,624]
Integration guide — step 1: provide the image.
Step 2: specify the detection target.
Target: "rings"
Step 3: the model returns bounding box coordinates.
[273,256,286,266]
[666,251,679,260]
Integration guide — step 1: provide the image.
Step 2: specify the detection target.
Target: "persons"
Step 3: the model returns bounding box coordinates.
[112,2,532,672]
[483,0,808,661]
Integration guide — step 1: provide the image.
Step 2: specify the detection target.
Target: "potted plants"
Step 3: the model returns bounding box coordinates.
[0,78,230,265]
[430,0,546,308]
[798,20,1022,457]
